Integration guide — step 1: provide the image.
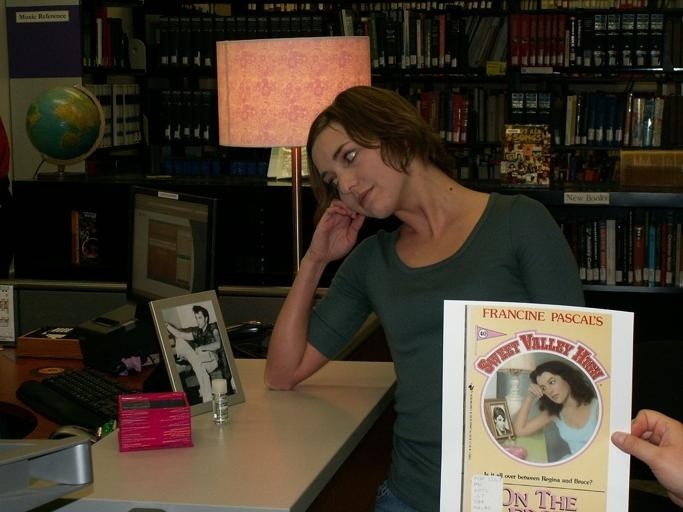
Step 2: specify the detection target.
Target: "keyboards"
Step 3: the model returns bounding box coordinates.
[44,368,132,416]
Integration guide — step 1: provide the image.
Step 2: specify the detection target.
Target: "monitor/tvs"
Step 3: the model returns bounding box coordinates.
[126,186,218,392]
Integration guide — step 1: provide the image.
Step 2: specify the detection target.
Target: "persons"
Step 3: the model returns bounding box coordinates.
[609,407,682,512]
[491,405,509,436]
[261,81,593,512]
[512,360,599,461]
[164,303,223,405]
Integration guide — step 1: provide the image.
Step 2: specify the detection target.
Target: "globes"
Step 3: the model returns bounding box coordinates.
[25,79,106,181]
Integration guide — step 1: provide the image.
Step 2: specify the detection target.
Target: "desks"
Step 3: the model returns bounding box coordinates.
[51,350,396,512]
[0,342,157,446]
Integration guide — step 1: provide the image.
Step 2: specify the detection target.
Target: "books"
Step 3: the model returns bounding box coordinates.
[82,15,145,151]
[331,0,682,290]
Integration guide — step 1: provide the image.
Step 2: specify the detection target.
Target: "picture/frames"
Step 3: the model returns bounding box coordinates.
[146,289,247,419]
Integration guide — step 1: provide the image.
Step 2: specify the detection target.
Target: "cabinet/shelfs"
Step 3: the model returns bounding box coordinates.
[0,0,682,365]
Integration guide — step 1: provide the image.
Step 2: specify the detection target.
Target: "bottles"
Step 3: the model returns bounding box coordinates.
[209,391,230,424]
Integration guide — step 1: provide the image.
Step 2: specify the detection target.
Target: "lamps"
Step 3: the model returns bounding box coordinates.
[210,36,373,283]
